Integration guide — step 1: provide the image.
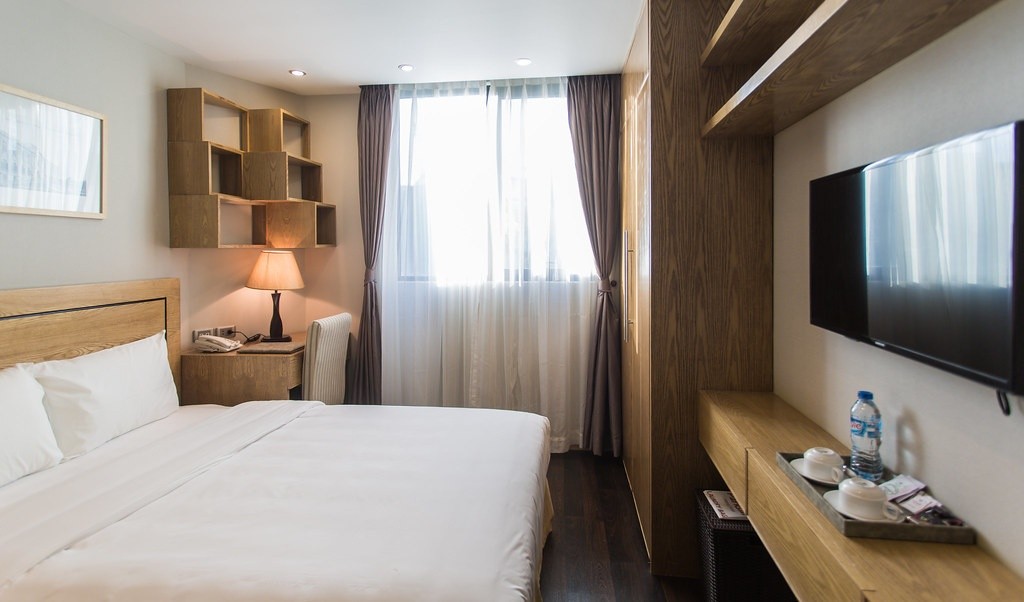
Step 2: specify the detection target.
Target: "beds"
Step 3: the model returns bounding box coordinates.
[0,276,556,602]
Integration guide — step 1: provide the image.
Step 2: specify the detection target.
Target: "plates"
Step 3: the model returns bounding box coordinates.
[823,490,907,524]
[790,458,856,486]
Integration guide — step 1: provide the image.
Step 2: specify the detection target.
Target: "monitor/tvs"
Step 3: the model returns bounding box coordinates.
[809,121,1024,396]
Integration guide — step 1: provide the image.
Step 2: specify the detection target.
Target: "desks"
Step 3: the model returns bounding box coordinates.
[180,332,351,406]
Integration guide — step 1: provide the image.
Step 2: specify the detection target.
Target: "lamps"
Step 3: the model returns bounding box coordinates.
[245,249,305,342]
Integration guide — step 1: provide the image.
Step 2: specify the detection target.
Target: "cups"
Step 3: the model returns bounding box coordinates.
[803,447,845,482]
[839,478,900,520]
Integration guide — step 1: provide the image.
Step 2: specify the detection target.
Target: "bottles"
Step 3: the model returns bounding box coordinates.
[849,391,883,480]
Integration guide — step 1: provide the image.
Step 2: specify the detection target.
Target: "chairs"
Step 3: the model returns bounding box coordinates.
[301,312,352,406]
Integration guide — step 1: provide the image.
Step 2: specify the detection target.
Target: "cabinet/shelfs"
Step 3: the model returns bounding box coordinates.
[167,87,337,248]
[687,0,1001,138]
[620,28,774,579]
[697,390,1024,602]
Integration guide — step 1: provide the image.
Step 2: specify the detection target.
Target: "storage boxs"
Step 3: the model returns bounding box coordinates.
[695,487,790,602]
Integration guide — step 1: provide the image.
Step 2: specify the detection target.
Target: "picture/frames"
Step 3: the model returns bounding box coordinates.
[0,84,108,221]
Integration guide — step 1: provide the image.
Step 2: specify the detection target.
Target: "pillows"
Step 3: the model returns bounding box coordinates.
[0,361,64,488]
[28,328,180,463]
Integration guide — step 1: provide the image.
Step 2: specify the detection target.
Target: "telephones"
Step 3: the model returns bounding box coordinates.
[195,335,243,352]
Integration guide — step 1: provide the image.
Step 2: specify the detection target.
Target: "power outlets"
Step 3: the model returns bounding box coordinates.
[215,325,236,338]
[193,328,214,342]
